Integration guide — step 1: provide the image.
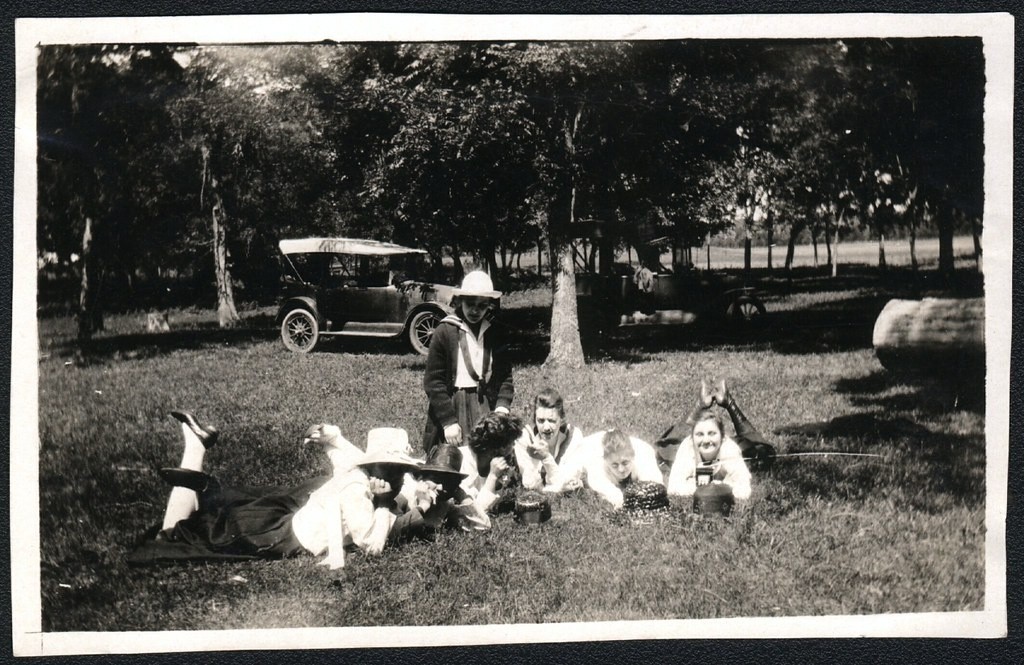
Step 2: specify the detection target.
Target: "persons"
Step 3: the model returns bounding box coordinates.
[632,260,654,294]
[422,268,515,453]
[130,388,668,564]
[328,257,343,278]
[658,378,778,498]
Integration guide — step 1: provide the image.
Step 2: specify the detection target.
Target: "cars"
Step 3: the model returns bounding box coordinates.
[275,234,462,359]
[550,217,767,343]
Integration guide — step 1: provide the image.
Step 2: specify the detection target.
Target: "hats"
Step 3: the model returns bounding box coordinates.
[452,270,502,299]
[355,428,422,470]
[420,444,469,480]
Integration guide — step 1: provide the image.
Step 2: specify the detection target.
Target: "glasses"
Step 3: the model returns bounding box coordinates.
[462,298,490,310]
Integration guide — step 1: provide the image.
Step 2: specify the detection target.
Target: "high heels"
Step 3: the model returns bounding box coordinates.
[160,467,221,496]
[171,409,220,449]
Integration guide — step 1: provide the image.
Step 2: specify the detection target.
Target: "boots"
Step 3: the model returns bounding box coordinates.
[684,381,714,428]
[714,379,747,426]
